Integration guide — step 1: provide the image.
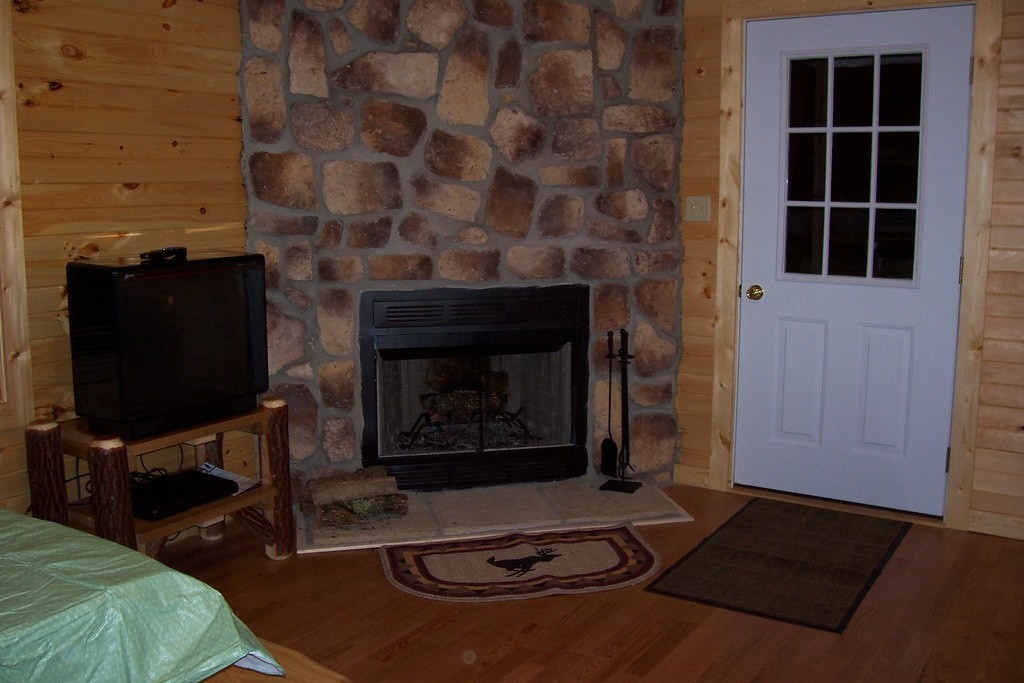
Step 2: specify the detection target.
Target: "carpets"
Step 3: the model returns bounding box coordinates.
[380,516,662,606]
[643,497,915,636]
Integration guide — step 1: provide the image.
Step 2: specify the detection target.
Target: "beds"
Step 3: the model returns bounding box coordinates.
[0,504,281,683]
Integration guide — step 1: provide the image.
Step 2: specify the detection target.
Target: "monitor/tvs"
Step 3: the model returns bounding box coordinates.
[66,250,269,443]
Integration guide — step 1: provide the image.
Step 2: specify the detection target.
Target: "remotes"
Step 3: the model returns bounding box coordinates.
[140,247,188,259]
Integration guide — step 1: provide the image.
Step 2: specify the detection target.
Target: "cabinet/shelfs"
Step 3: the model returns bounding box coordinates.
[25,396,296,571]
